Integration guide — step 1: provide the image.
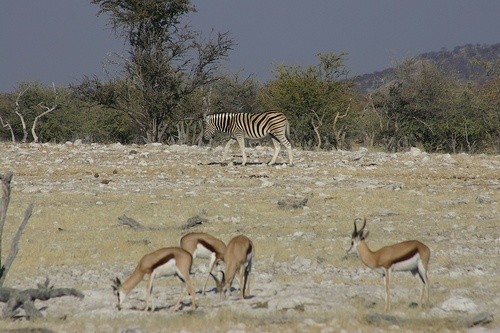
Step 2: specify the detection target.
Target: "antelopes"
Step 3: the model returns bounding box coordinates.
[180,232,226,295]
[346,218,431,309]
[110,247,197,312]
[209,235,256,302]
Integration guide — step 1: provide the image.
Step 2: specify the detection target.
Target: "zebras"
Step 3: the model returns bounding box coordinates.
[202,109,295,166]
